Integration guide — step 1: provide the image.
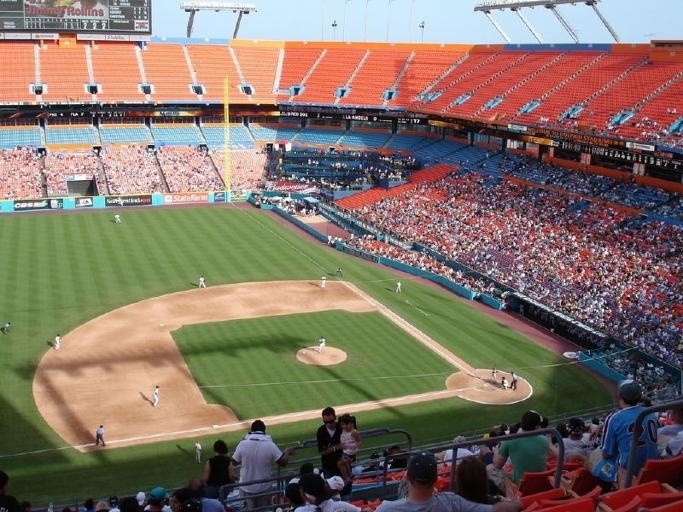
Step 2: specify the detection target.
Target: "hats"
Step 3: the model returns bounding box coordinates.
[149,488,170,500]
[522,410,543,426]
[286,464,345,500]
[407,451,438,483]
[618,379,643,403]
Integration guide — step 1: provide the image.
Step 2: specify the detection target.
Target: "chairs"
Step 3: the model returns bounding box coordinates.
[332,447,682,512]
[500,211,682,403]
[344,211,499,291]
[0,42,682,210]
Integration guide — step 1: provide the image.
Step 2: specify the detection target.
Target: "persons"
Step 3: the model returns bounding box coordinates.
[205,401,683,511]
[4,322,11,332]
[195,441,201,464]
[0,139,334,218]
[199,276,206,288]
[112,214,121,224]
[55,334,61,350]
[504,298,532,315]
[152,385,161,406]
[19,479,224,512]
[96,424,105,445]
[320,275,326,288]
[491,368,517,390]
[318,336,326,353]
[532,297,682,400]
[2,473,19,512]
[333,104,683,297]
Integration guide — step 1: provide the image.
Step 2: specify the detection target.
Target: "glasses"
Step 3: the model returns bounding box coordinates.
[323,417,336,424]
